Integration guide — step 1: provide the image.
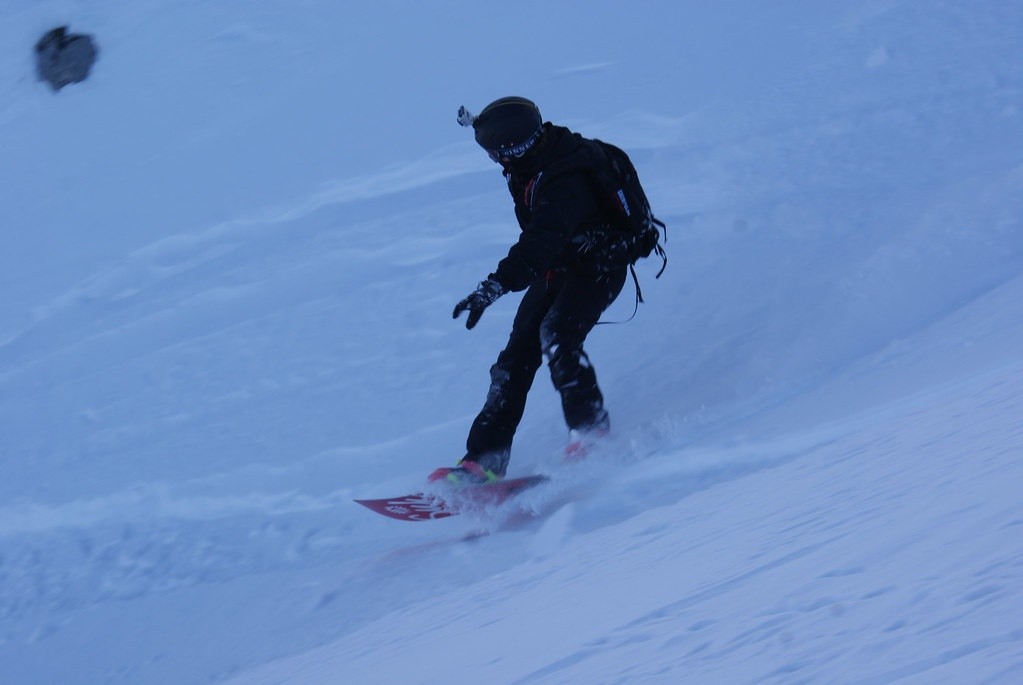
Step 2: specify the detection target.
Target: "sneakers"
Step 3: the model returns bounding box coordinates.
[426,449,512,489]
[554,409,612,463]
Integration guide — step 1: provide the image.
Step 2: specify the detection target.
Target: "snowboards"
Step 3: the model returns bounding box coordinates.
[351,472,556,522]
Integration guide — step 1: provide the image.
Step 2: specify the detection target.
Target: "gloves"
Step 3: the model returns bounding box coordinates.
[451,273,510,330]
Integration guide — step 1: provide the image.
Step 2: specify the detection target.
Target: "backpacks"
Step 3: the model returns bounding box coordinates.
[544,130,668,303]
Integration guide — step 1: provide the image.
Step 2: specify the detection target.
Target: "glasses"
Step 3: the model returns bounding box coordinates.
[488,149,500,163]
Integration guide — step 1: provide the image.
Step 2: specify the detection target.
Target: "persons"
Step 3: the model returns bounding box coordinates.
[429,95,661,483]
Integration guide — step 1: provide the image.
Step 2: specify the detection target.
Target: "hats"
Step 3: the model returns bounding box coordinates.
[475,96,543,151]
